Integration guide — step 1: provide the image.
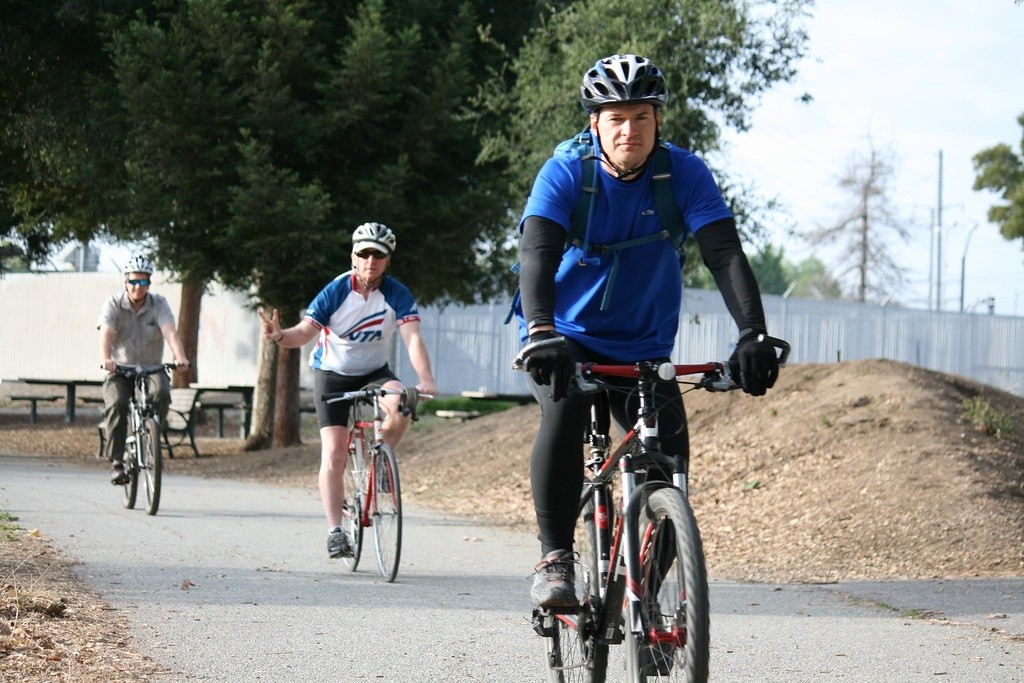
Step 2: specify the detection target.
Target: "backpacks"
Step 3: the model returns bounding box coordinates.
[504,122,690,325]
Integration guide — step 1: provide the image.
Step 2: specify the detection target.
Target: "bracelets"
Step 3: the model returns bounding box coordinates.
[274,331,284,343]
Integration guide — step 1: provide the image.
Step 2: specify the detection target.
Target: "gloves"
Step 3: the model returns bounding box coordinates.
[530,330,575,403]
[729,327,779,397]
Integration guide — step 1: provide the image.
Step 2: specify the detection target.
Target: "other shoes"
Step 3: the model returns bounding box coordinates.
[110,463,125,483]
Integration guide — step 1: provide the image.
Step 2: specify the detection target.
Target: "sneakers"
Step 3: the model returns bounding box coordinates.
[327,527,353,558]
[376,451,393,493]
[525,549,581,607]
[642,602,674,676]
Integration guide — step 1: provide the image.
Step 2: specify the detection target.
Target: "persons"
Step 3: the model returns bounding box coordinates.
[256,223,435,559]
[96,255,189,485]
[514,55,780,674]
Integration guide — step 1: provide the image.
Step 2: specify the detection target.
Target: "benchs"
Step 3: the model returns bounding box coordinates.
[98,385,205,462]
[80,395,104,406]
[201,401,317,413]
[433,407,480,419]
[9,392,61,424]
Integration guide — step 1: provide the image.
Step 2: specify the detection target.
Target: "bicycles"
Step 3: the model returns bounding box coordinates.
[321,382,433,582]
[101,361,178,516]
[510,360,738,683]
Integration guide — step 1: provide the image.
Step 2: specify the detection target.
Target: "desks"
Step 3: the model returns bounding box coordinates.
[187,385,307,437]
[25,379,107,424]
[459,389,534,405]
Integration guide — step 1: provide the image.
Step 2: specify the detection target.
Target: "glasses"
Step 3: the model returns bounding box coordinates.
[127,276,149,287]
[354,248,387,259]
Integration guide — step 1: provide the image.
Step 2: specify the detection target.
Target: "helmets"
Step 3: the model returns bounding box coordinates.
[352,223,396,256]
[124,255,153,274]
[580,54,669,111]
